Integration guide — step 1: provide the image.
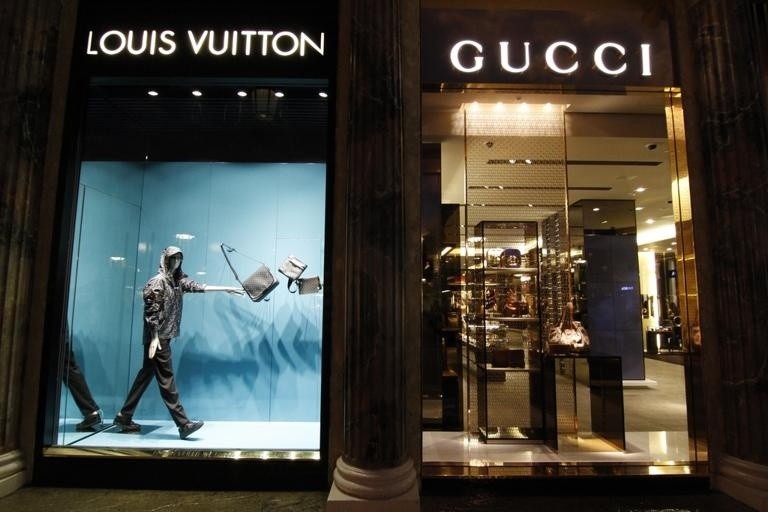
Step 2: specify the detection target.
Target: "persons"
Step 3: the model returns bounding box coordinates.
[112,243,245,440]
[63,322,101,431]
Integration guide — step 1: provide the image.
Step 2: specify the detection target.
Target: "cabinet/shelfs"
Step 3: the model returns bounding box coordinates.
[440,204,546,442]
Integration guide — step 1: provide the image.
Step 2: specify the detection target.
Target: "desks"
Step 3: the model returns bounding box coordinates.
[530,348,626,452]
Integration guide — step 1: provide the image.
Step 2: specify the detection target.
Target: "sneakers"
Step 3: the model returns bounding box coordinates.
[113,414,142,431]
[178,419,205,440]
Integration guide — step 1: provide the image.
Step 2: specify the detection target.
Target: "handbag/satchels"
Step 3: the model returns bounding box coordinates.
[278,254,309,292]
[241,262,280,302]
[297,275,322,295]
